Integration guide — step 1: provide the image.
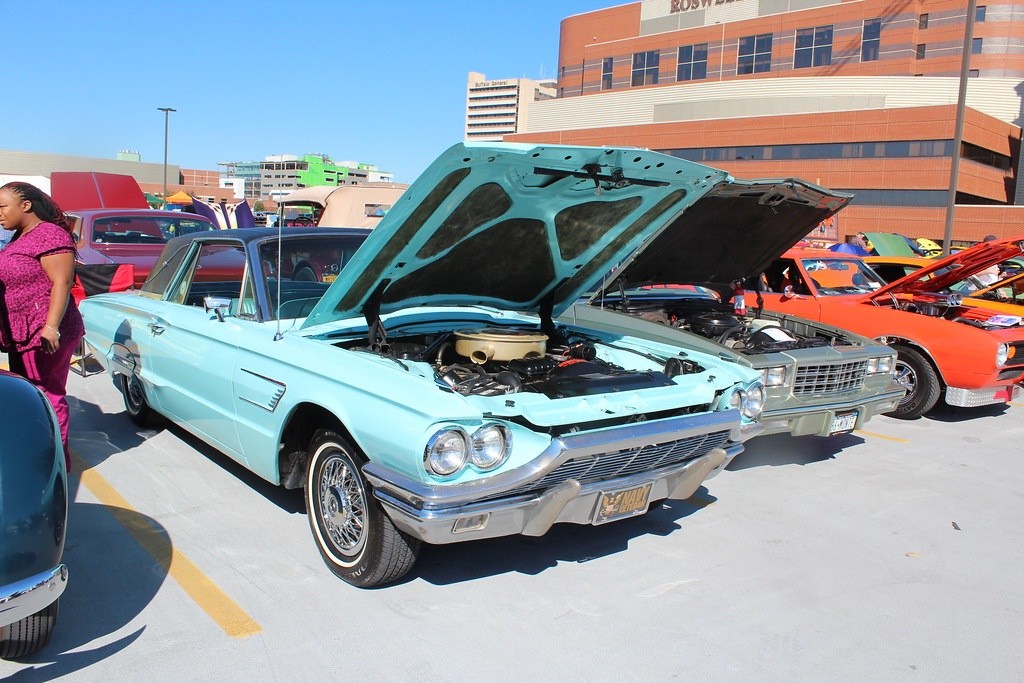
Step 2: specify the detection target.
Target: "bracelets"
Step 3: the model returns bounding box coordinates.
[44,323,61,338]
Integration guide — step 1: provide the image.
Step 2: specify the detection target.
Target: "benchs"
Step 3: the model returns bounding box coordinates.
[190,280,329,306]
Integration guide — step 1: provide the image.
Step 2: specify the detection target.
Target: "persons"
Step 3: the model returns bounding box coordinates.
[0,182,85,474]
[851,232,867,248]
[975,234,1007,295]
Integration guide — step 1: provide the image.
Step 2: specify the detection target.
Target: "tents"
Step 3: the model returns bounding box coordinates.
[144,189,194,210]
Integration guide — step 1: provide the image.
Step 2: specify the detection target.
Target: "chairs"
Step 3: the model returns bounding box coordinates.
[275,290,324,304]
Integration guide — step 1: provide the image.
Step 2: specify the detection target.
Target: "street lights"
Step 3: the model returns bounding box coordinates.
[157,106,176,235]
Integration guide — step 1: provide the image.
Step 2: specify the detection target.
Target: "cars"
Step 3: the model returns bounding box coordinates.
[42,170,274,299]
[573,174,907,440]
[0,362,72,660]
[73,139,770,593]
[189,183,412,282]
[642,232,1024,421]
